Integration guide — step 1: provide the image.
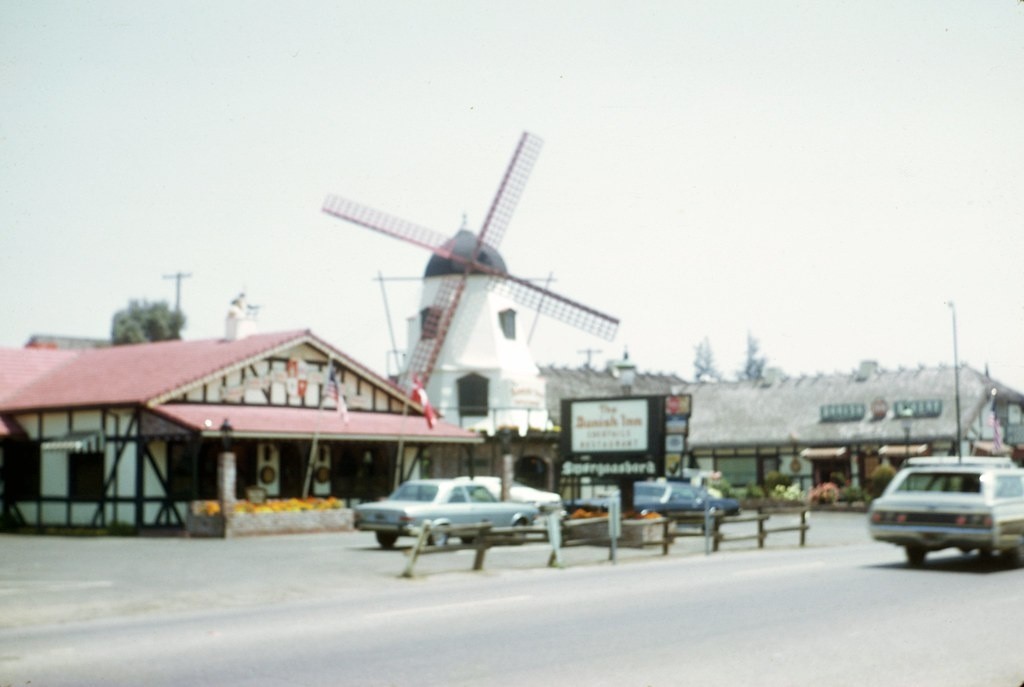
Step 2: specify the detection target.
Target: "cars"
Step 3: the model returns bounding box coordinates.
[571,477,740,527]
[867,453,1024,565]
[351,476,532,551]
[465,473,564,518]
